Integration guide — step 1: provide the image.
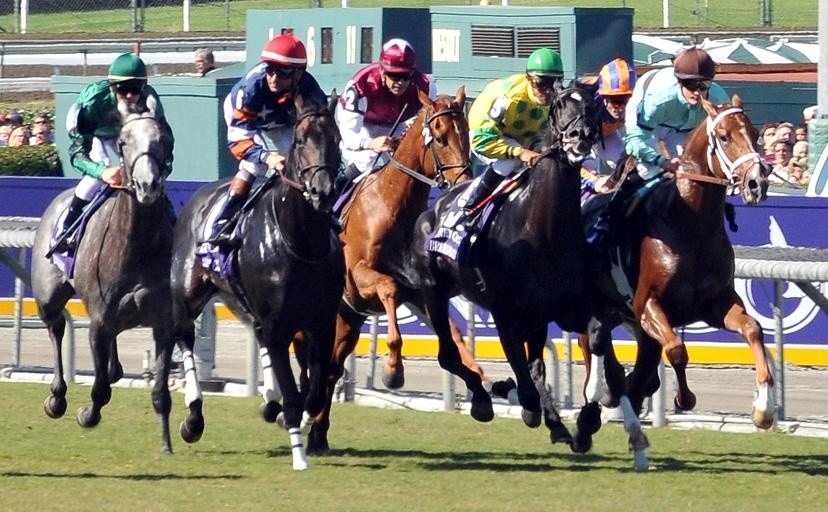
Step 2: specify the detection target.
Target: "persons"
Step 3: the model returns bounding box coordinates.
[574,59,637,209]
[608,49,730,212]
[195,48,217,77]
[757,105,822,188]
[0,109,49,147]
[460,47,566,230]
[210,34,344,246]
[328,38,436,203]
[50,52,174,249]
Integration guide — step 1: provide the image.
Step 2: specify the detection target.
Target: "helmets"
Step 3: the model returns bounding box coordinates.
[108,53,148,84]
[260,33,307,68]
[674,48,714,80]
[526,49,564,78]
[599,58,635,96]
[380,39,417,73]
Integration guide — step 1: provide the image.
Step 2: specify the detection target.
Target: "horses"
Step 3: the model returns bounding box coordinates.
[588,90,778,432]
[168,86,349,476]
[403,74,620,449]
[29,90,182,458]
[287,83,473,449]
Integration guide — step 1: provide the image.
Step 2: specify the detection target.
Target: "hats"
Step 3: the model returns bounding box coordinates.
[6,113,23,125]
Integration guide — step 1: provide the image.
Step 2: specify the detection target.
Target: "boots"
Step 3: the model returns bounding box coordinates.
[585,177,638,249]
[462,162,506,234]
[208,191,250,250]
[331,163,363,208]
[55,192,91,254]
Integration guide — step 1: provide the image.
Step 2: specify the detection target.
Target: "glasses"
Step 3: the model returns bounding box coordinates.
[263,66,305,79]
[678,77,713,91]
[111,81,144,96]
[527,74,555,92]
[601,95,631,106]
[381,68,413,82]
